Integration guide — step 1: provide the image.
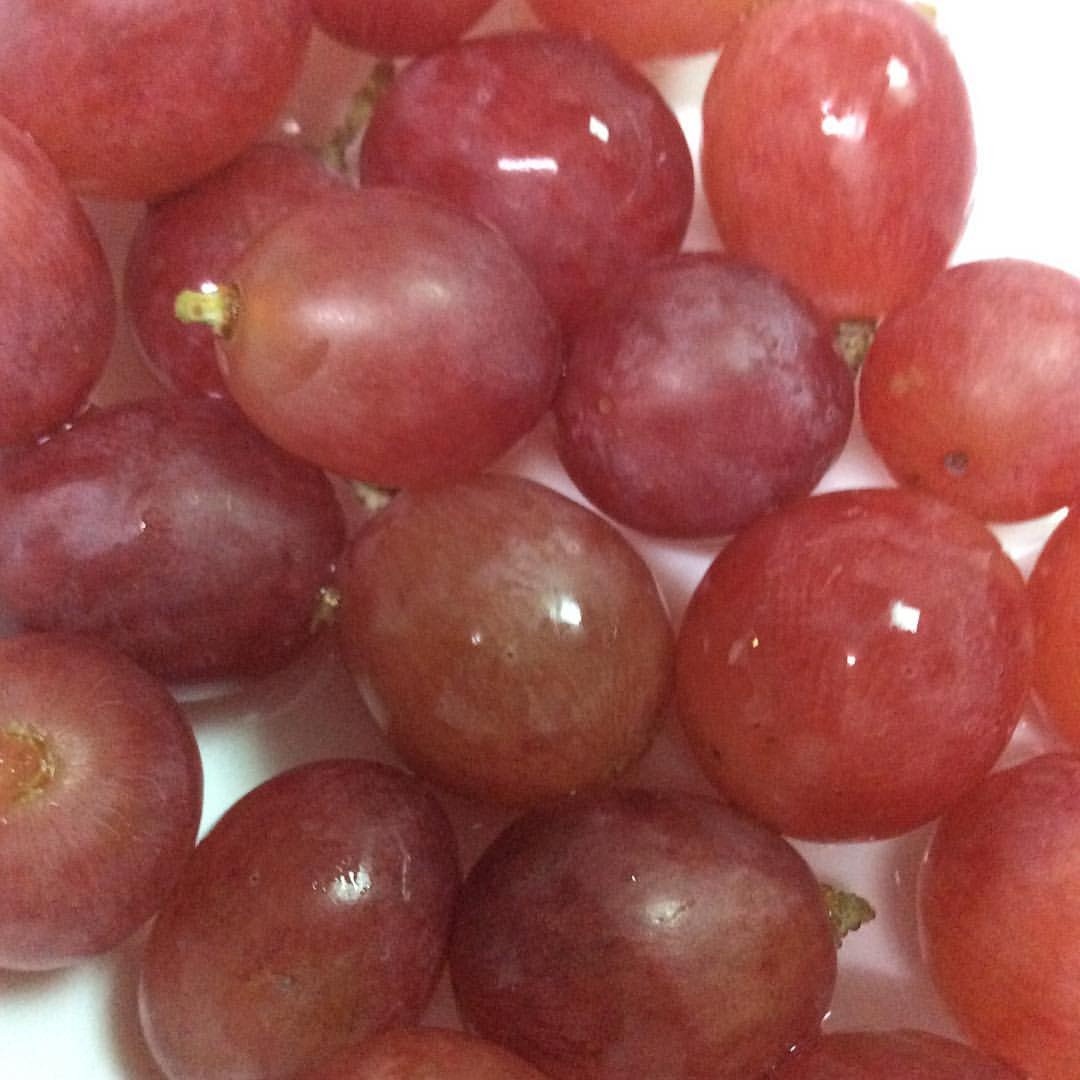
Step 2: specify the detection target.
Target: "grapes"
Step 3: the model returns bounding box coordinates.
[0,1,1080,1080]
